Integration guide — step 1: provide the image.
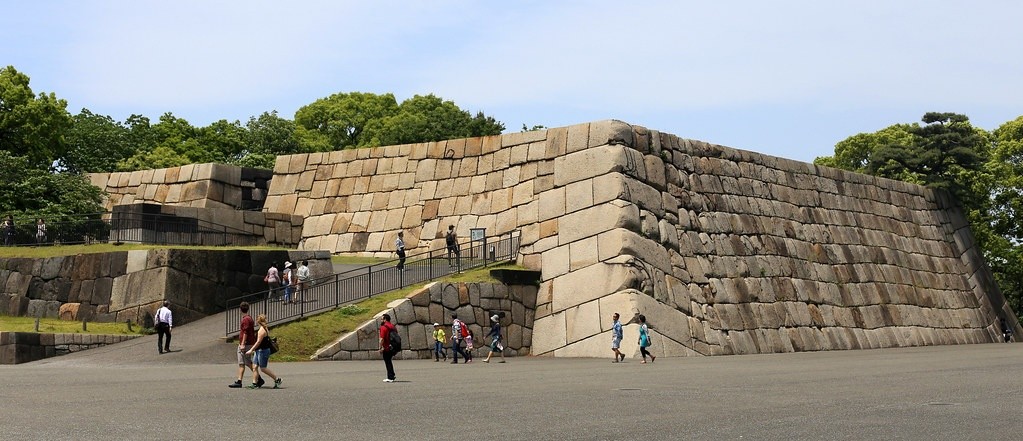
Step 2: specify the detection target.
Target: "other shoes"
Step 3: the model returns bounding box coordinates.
[247,383,260,390]
[621,354,625,361]
[228,380,242,388]
[164,348,170,352]
[652,356,656,362]
[450,360,458,364]
[465,357,470,363]
[255,375,265,387]
[482,359,489,363]
[612,360,618,363]
[159,351,164,354]
[498,360,505,363]
[640,361,646,363]
[273,377,283,389]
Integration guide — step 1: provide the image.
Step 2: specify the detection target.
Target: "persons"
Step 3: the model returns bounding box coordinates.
[611,313,625,363]
[268,259,309,305]
[638,314,657,364]
[446,225,461,268]
[464,330,473,363]
[433,322,448,361]
[450,313,470,364]
[38,218,46,246]
[395,231,406,271]
[155,300,173,354]
[482,315,506,363]
[379,314,397,382]
[245,314,282,389]
[228,302,265,388]
[4,215,14,247]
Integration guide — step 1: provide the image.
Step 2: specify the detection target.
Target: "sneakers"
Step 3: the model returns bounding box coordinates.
[383,376,396,383]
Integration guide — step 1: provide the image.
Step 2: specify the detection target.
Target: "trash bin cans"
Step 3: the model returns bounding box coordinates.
[16,225,36,244]
[490,246,495,260]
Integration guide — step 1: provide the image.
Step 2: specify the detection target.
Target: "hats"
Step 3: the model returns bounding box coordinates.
[284,261,293,268]
[434,323,440,327]
[449,224,455,227]
[490,314,500,323]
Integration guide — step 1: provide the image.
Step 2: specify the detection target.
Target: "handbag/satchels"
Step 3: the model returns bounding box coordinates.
[282,269,292,283]
[268,336,280,355]
[156,322,164,334]
[264,275,270,282]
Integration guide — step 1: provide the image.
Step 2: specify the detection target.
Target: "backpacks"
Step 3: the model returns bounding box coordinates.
[456,320,471,338]
[382,324,402,353]
[446,231,454,246]
